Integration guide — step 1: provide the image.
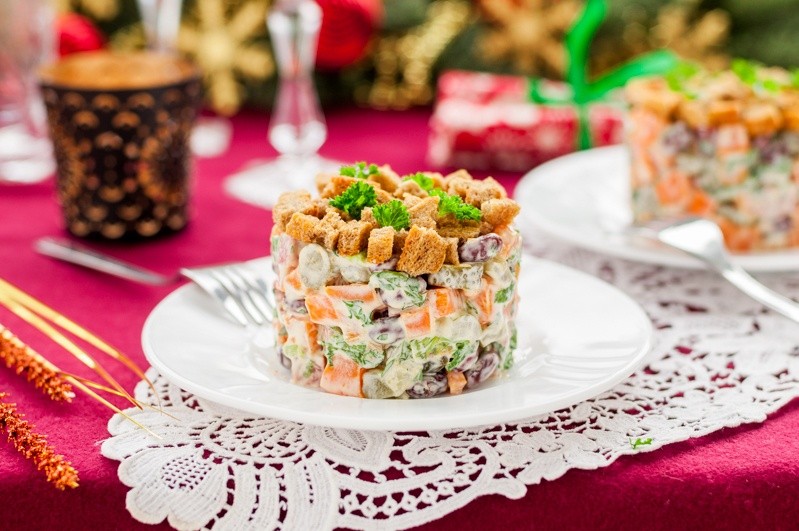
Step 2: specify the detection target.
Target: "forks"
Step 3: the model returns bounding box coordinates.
[624,214,799,323]
[32,238,280,330]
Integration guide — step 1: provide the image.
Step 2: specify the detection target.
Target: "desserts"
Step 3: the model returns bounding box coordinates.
[271,163,522,396]
[621,58,799,253]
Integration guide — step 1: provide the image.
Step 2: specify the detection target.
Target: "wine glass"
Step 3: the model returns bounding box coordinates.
[222,1,357,209]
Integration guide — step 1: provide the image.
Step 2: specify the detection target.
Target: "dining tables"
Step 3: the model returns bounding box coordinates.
[0,109,799,531]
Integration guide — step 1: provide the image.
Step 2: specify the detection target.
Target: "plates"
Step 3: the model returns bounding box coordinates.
[138,252,657,434]
[513,144,798,272]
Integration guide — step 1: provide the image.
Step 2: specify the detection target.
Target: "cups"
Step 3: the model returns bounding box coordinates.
[31,49,211,247]
